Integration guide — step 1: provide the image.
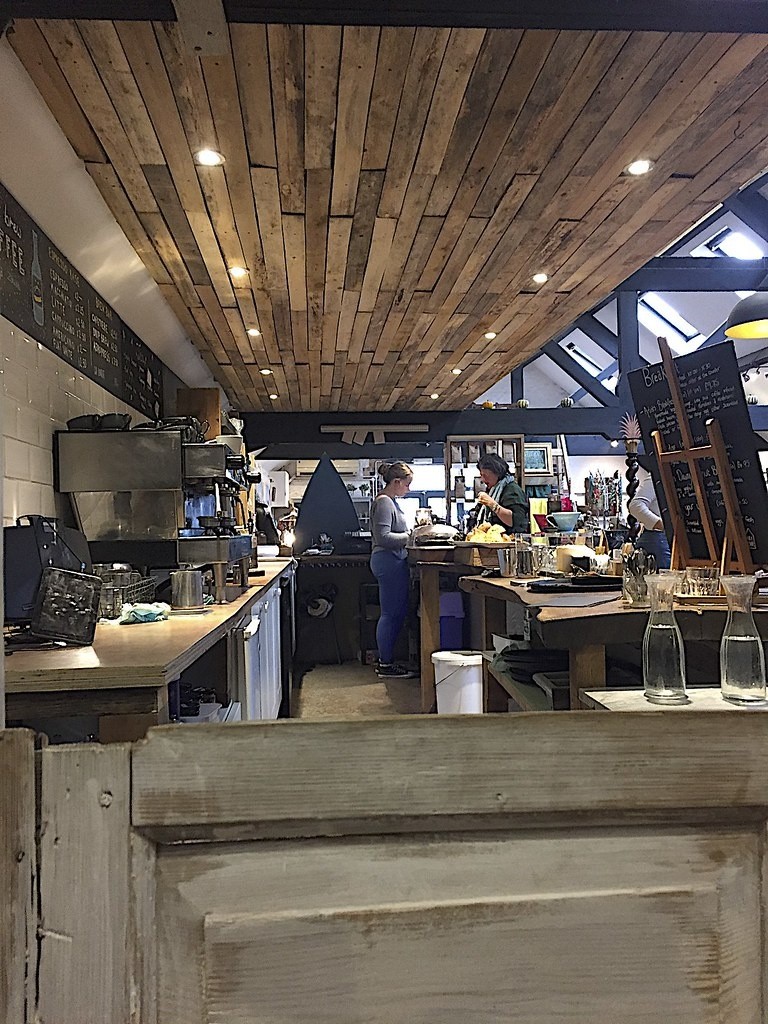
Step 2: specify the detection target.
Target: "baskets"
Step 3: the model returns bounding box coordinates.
[98,576,158,619]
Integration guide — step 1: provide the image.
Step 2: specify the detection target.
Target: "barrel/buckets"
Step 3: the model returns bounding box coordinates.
[170,571,204,610]
[432,651,483,714]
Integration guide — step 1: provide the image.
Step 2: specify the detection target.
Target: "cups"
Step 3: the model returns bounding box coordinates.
[518,547,537,578]
[545,511,582,531]
[497,549,518,577]
[659,569,687,595]
[687,567,712,596]
[712,568,719,595]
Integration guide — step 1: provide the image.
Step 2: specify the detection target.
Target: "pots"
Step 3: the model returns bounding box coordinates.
[196,516,231,527]
[66,414,101,429]
[101,413,132,428]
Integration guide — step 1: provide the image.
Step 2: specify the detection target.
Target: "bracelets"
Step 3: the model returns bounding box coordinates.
[494,504,501,513]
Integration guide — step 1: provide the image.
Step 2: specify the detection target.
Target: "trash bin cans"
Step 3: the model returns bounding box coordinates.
[431,650,482,714]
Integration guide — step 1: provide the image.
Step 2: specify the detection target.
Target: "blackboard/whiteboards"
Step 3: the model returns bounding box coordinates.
[626,340,768,589]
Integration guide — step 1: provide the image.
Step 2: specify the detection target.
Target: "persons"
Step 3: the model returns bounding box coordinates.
[629,473,672,572]
[369,461,417,678]
[472,453,531,538]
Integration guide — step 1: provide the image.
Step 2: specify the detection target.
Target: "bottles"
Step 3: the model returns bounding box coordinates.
[548,489,561,528]
[717,575,766,701]
[561,490,573,512]
[642,574,686,698]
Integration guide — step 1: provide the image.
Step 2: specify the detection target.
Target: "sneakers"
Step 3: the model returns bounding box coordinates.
[375,658,414,679]
